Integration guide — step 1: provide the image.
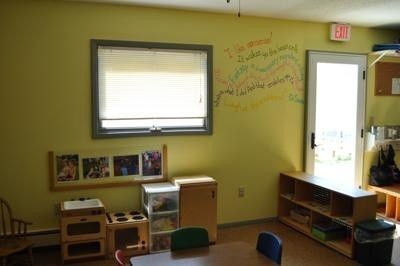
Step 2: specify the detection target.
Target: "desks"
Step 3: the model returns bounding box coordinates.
[130,241,279,266]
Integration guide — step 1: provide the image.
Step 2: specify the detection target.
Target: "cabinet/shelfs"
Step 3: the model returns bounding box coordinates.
[366,184,400,228]
[59,198,106,262]
[168,173,218,244]
[277,169,379,261]
[140,181,179,254]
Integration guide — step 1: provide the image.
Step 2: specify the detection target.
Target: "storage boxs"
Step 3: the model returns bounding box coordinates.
[312,220,347,243]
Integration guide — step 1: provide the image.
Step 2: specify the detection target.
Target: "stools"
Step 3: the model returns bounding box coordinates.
[103,210,149,259]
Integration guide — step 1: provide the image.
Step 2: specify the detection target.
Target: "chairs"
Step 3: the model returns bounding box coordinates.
[255,232,283,266]
[170,226,209,252]
[0,197,35,266]
[115,249,128,266]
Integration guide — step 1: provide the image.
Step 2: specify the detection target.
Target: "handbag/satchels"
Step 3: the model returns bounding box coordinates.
[369,144,400,187]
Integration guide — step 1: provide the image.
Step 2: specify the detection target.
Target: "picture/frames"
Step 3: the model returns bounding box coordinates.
[140,149,164,181]
[112,154,141,179]
[54,152,81,183]
[80,156,112,181]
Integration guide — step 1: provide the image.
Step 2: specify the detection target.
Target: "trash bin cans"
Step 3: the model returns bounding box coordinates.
[352,220,396,266]
